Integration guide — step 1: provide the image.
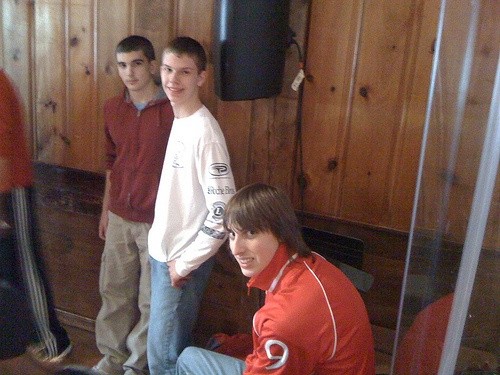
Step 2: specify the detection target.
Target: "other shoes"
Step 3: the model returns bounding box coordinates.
[27,336,72,371]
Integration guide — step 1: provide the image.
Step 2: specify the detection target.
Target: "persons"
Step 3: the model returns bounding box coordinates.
[147,37,236,375]
[0,68,71,362]
[175,183,375,375]
[92,35,175,375]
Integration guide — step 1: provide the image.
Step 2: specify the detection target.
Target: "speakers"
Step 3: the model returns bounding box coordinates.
[214,0,290,101]
